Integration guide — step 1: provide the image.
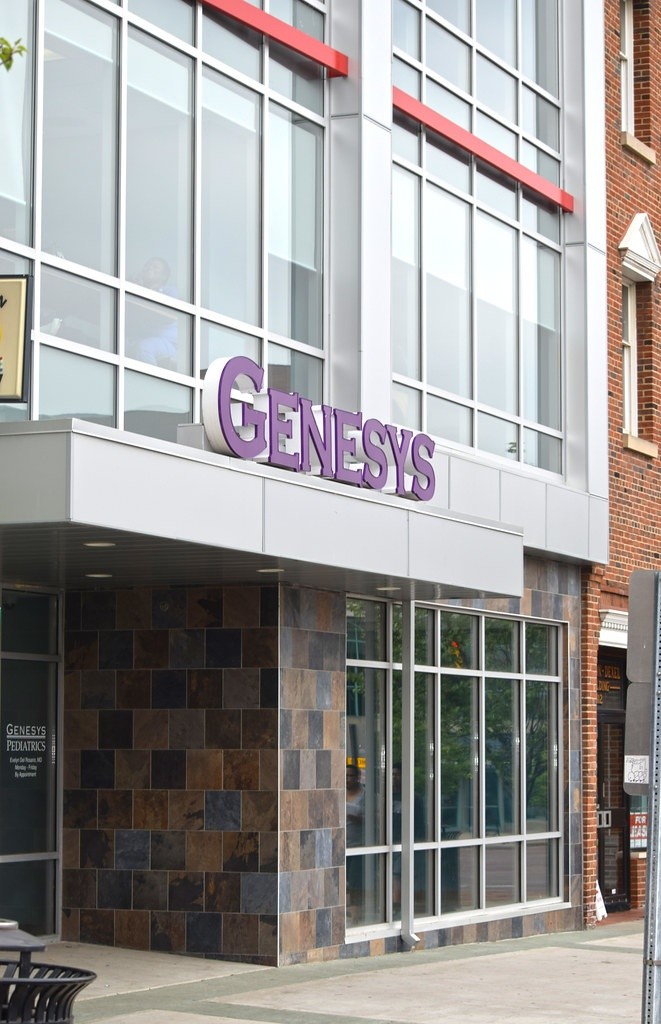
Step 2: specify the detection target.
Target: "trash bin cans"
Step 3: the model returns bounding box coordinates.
[0,919,97,1024]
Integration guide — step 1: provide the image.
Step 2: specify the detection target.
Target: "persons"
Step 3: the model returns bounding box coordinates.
[345,764,402,927]
